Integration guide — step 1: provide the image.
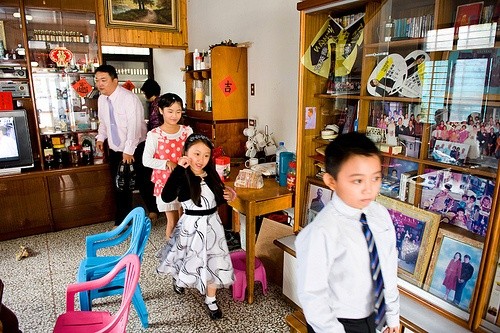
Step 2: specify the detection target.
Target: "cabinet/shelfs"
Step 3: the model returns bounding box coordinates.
[181,46,248,165]
[273,0,498,333]
[0,0,115,242]
[420,22,495,126]
[101,44,156,132]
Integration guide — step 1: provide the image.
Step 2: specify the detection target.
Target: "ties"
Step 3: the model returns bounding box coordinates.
[107,98,121,147]
[359,213,387,331]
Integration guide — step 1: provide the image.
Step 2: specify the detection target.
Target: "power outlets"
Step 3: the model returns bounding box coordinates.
[250,83,255,96]
[249,119,256,126]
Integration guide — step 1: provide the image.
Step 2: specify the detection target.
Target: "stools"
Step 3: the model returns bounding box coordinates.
[229,251,268,303]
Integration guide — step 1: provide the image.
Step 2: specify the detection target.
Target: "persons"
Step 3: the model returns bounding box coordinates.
[310,55,500,304]
[93,64,236,320]
[294,132,399,333]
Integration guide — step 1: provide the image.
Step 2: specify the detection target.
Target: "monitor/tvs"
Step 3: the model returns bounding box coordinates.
[0,109,34,176]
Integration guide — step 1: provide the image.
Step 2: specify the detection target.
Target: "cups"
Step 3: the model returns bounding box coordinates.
[245,158,258,169]
[279,152,293,186]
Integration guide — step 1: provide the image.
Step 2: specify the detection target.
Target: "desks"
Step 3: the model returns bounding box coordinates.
[220,162,295,306]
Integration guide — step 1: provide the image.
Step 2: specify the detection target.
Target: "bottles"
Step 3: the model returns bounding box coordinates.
[33,28,90,44]
[286,162,297,192]
[90,108,98,130]
[193,49,205,70]
[275,142,287,176]
[216,156,231,182]
[42,135,94,171]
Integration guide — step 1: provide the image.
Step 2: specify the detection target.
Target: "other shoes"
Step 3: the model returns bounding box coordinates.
[205,300,222,319]
[116,162,137,192]
[149,213,158,225]
[173,277,185,295]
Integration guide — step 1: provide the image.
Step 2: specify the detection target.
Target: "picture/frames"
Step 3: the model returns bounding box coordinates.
[367,99,499,239]
[300,175,333,228]
[376,190,443,289]
[422,225,487,313]
[103,0,181,33]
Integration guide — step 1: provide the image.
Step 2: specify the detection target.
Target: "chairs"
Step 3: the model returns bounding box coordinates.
[52,206,153,333]
